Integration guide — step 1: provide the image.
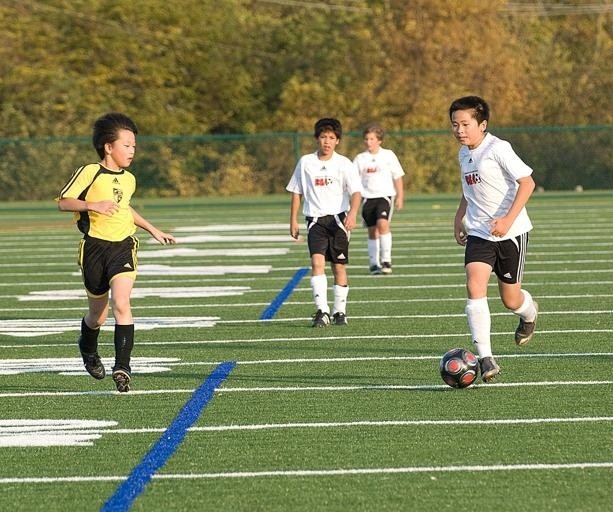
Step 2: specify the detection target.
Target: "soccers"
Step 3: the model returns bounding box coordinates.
[439,348,477,389]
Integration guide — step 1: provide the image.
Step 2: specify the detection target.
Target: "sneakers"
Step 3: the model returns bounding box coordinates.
[310,308,331,328]
[78,334,106,380]
[111,363,132,392]
[478,356,502,384]
[369,264,382,275]
[514,301,539,347]
[381,261,393,274]
[332,311,348,326]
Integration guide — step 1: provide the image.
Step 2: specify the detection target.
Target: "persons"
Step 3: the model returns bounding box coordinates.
[54,113,178,392]
[450,96,539,381]
[351,126,406,273]
[285,116,362,325]
